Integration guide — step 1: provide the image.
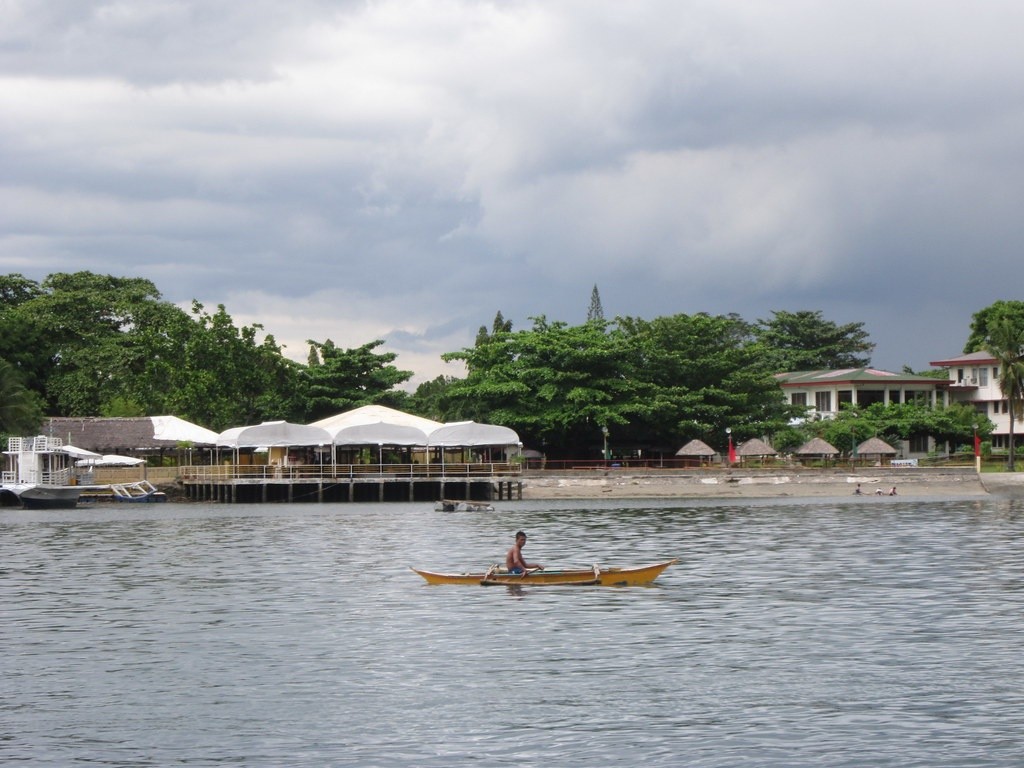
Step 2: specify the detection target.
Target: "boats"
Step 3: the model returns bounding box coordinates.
[435,500,493,511]
[9,487,83,509]
[410,559,679,589]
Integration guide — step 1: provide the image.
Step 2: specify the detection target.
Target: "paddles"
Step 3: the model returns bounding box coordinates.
[520,567,540,581]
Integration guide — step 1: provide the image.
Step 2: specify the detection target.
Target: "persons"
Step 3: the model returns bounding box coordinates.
[856,484,863,493]
[889,487,896,496]
[506,530,544,579]
[875,488,883,495]
